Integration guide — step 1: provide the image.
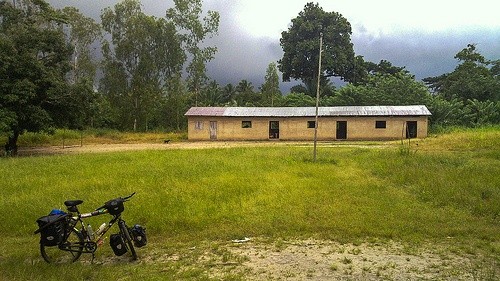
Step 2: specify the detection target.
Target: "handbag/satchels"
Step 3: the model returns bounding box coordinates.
[35,214,65,247]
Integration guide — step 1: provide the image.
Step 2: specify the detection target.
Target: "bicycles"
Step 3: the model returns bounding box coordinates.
[34,193,138,265]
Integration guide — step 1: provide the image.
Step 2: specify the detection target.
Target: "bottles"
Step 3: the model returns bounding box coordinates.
[97,223,106,235]
[87,224,95,240]
[97,237,108,246]
